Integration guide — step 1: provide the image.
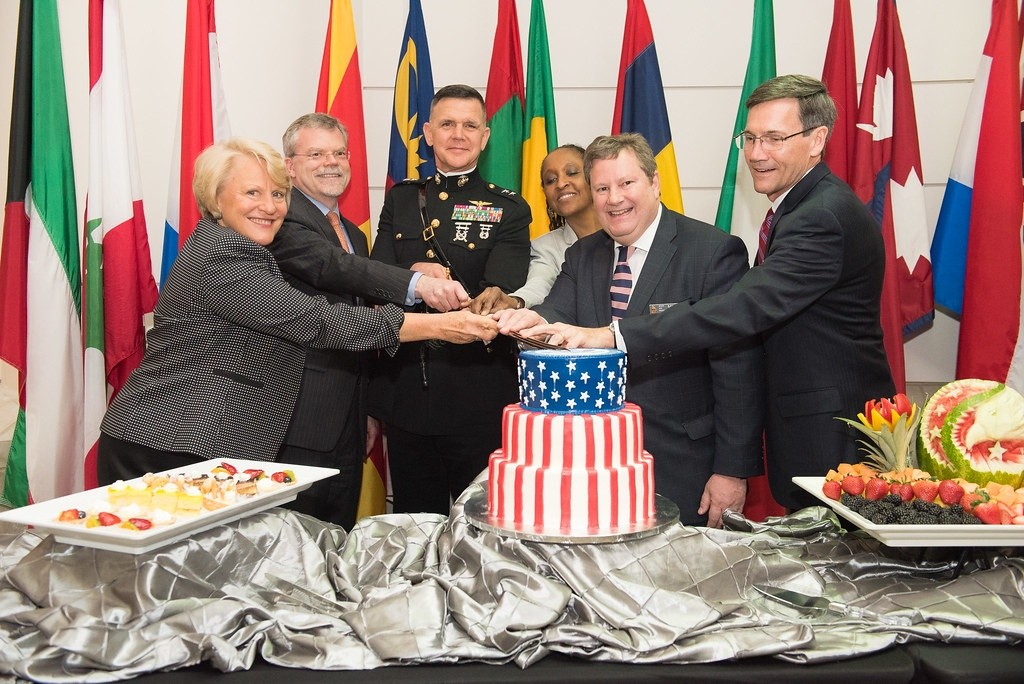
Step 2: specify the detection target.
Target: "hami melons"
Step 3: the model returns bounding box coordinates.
[825,463,1024,510]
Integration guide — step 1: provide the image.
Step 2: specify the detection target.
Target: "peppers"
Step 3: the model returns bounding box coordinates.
[858,393,916,432]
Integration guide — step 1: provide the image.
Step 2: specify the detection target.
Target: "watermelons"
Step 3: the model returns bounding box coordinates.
[916,378,1024,488]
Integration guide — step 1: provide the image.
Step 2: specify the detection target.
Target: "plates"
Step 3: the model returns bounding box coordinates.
[0,456,340,554]
[789,476,1024,551]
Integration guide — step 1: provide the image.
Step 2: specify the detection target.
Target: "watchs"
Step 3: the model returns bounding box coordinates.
[609,323,617,349]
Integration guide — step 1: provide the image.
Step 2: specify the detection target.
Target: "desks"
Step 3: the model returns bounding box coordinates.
[127,646,914,684]
[903,638,1024,684]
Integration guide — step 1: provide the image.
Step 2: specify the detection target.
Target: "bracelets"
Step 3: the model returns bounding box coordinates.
[517,301,520,308]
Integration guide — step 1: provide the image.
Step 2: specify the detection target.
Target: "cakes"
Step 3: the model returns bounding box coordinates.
[487,348,654,534]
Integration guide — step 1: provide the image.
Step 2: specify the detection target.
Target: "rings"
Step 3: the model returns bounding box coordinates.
[476,337,479,340]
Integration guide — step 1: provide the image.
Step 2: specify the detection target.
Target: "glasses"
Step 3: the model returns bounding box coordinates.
[733,125,824,150]
[289,150,350,161]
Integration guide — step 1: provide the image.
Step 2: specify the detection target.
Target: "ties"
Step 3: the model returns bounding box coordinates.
[326,211,350,254]
[609,245,637,321]
[758,207,774,265]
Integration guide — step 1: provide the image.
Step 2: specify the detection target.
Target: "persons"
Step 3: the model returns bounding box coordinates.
[98,138,498,487]
[518,75,896,531]
[265,112,468,534]
[363,85,533,516]
[493,132,762,533]
[459,144,603,317]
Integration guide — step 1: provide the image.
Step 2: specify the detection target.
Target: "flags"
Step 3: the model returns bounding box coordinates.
[712,0,1024,517]
[387,0,438,196]
[0,0,83,511]
[476,1,557,239]
[161,1,235,291]
[315,0,386,528]
[611,0,684,217]
[83,0,162,495]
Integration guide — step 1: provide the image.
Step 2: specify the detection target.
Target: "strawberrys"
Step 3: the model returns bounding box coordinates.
[221,462,237,475]
[98,511,121,527]
[129,518,152,530]
[271,472,288,483]
[823,474,1003,525]
[59,509,79,521]
[242,469,264,479]
